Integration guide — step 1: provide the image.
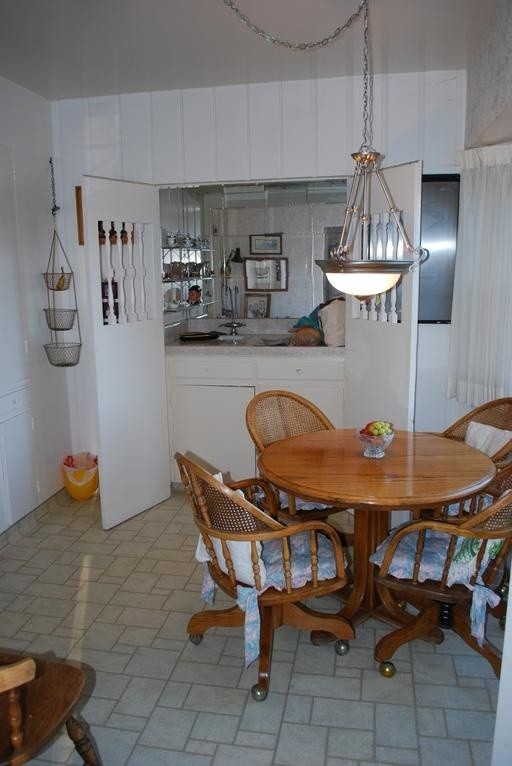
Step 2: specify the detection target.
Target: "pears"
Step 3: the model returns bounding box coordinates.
[57,277,64,289]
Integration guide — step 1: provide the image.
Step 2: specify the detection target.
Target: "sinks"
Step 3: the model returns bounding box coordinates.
[216,334,250,341]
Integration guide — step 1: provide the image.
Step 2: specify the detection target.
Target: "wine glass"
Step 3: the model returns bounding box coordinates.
[353,426,395,458]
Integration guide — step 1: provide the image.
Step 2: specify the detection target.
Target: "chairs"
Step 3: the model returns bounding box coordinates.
[165,446,355,699]
[0,642,114,766]
[239,381,357,547]
[425,395,512,525]
[371,485,512,684]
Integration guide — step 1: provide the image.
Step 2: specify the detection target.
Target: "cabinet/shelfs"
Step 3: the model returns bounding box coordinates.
[164,246,218,310]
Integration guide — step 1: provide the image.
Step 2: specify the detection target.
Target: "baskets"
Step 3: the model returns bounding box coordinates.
[44,342,81,367]
[43,272,72,291]
[44,308,76,331]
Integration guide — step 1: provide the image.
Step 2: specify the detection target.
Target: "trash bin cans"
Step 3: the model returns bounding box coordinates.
[63,452,99,502]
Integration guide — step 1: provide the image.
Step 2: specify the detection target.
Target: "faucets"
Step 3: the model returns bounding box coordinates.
[218,322,247,335]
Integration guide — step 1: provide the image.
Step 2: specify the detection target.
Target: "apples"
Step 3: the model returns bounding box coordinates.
[360,423,383,447]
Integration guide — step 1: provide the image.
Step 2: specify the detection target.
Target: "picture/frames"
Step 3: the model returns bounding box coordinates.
[73,184,136,246]
[242,292,271,317]
[242,256,289,291]
[248,233,283,254]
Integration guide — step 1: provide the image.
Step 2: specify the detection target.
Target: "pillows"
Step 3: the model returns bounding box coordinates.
[461,417,511,460]
[451,513,504,584]
[193,469,263,587]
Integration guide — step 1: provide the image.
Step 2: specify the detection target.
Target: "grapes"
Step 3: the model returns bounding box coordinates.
[371,421,393,437]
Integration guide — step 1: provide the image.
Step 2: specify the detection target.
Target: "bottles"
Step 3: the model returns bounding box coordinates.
[166,234,209,248]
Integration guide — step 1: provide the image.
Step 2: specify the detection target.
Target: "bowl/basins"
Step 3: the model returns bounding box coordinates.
[166,302,180,310]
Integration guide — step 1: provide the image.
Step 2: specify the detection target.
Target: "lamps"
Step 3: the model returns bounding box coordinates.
[222,242,244,269]
[219,0,427,301]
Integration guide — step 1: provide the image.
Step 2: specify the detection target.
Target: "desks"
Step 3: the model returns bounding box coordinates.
[256,425,497,643]
